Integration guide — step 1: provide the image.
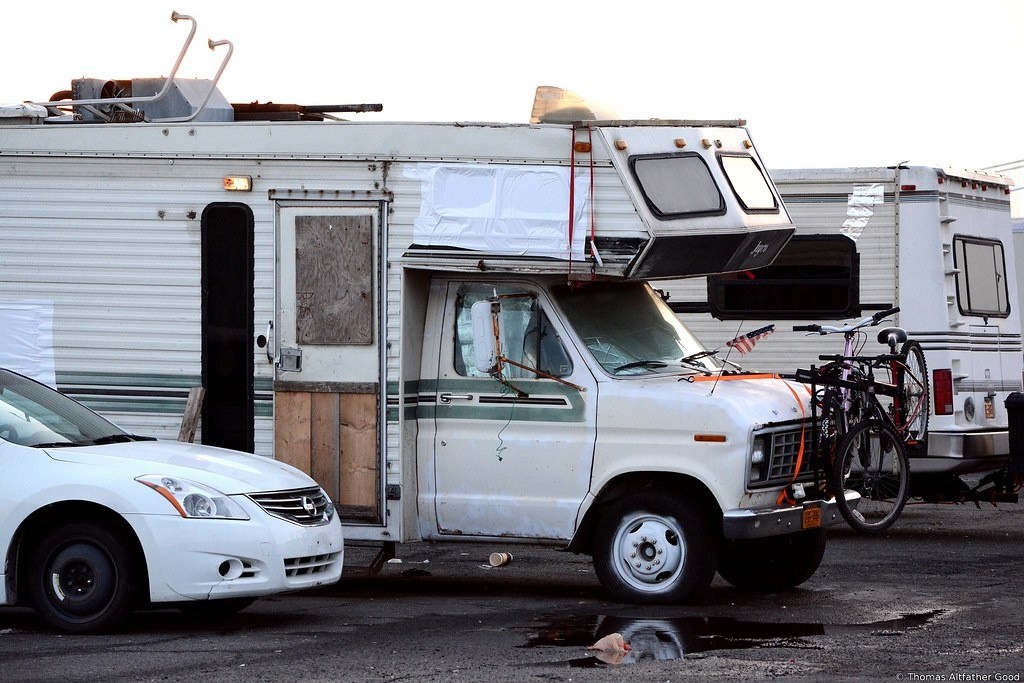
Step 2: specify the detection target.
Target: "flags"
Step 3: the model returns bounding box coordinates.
[726,324,776,357]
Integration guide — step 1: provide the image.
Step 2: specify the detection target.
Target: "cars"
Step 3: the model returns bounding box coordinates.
[0,367,345,634]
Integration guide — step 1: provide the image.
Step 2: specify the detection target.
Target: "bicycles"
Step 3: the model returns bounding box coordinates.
[792,306,902,492]
[818,327,930,538]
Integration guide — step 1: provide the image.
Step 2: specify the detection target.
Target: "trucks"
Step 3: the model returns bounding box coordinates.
[1,10,861,608]
[646,165,1022,476]
